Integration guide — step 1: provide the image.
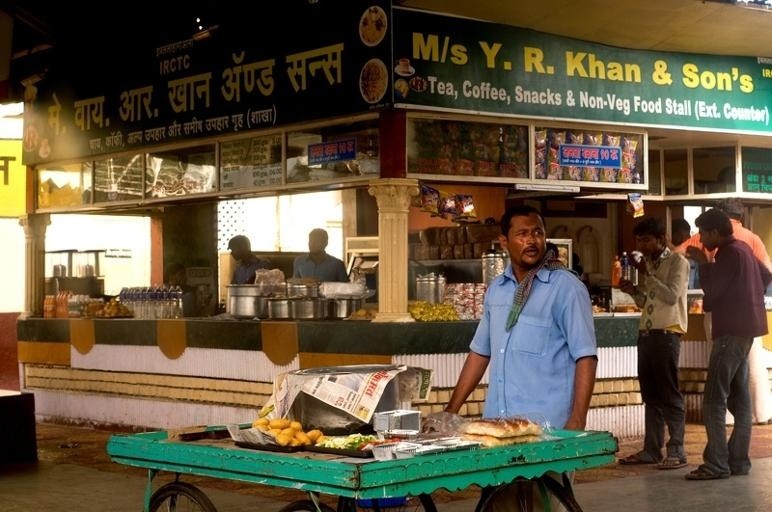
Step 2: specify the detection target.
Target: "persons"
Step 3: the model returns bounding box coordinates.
[616,216,691,470]
[292,227,350,282]
[683,208,772,481]
[444,201,598,512]
[225,234,277,283]
[673,198,772,427]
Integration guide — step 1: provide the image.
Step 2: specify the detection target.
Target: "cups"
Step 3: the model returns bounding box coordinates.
[399,59,410,73]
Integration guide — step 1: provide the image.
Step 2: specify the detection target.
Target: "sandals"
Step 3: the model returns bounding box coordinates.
[684,462,732,481]
[617,448,688,470]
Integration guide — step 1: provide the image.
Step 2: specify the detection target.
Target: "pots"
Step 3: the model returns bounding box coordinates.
[228,282,362,321]
[290,367,399,436]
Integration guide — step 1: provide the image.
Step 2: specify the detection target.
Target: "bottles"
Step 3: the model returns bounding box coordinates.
[43,285,183,320]
[613,251,631,286]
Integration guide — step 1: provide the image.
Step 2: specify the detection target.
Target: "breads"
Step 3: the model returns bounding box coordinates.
[255,418,326,446]
[462,417,541,448]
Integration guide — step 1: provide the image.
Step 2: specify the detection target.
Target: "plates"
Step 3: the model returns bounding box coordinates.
[359,7,389,104]
[395,66,416,76]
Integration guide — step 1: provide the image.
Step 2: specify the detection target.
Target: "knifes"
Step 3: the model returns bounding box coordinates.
[181,426,253,442]
